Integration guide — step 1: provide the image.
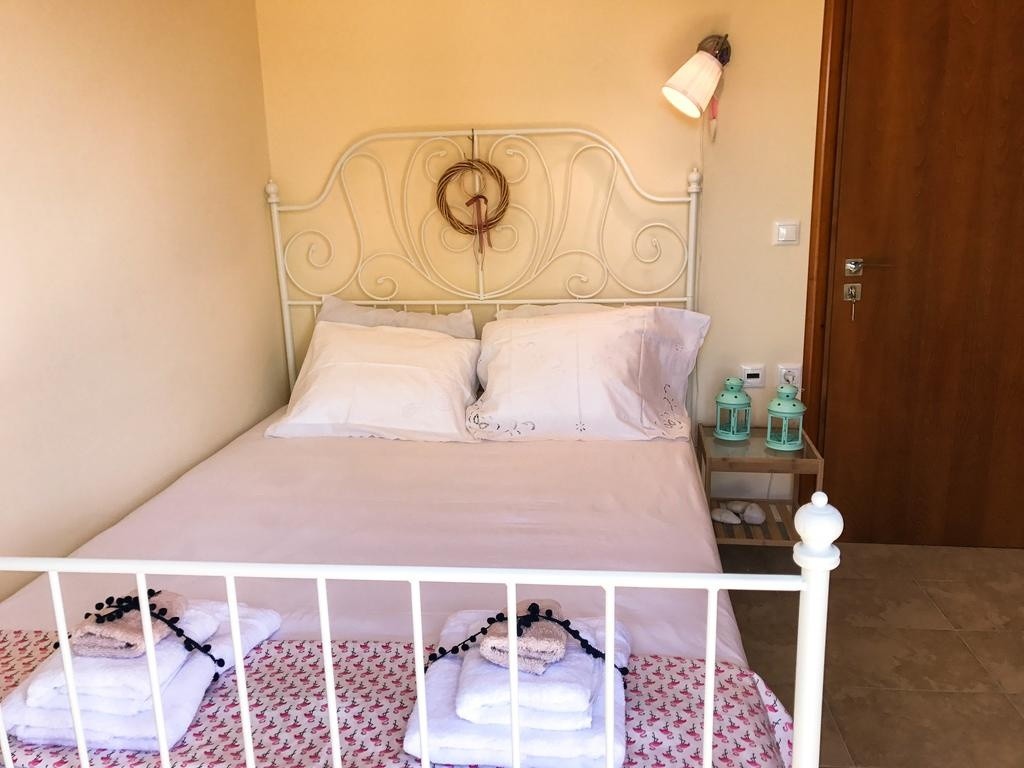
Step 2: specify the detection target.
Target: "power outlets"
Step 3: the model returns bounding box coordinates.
[778,364,801,390]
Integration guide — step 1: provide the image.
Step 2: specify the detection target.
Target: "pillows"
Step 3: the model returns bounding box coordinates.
[265,294,711,445]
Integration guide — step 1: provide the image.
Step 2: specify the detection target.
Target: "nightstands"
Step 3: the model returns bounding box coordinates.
[696,424,824,547]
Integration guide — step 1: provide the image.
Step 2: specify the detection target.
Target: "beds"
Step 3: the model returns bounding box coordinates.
[0,123,846,768]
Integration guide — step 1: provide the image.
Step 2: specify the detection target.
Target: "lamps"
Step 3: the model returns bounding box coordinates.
[662,33,733,121]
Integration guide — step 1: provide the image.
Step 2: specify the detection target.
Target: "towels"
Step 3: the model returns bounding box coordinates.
[456,616,602,732]
[0,598,284,752]
[69,588,189,660]
[401,610,632,768]
[478,598,568,675]
[23,608,219,717]
